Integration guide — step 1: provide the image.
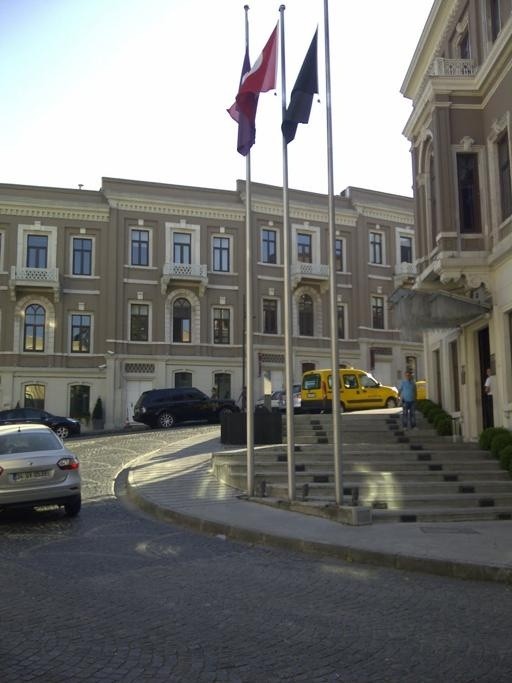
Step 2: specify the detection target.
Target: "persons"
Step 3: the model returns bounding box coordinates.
[397,371,418,430]
[484,368,493,428]
[237,386,246,411]
[210,387,217,400]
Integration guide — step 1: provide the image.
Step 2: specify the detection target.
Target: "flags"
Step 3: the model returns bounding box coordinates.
[281,24,319,144]
[235,18,280,122]
[226,44,256,157]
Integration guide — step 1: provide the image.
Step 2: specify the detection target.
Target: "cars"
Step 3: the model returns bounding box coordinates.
[132,387,241,428]
[255,368,400,413]
[0,408,82,516]
